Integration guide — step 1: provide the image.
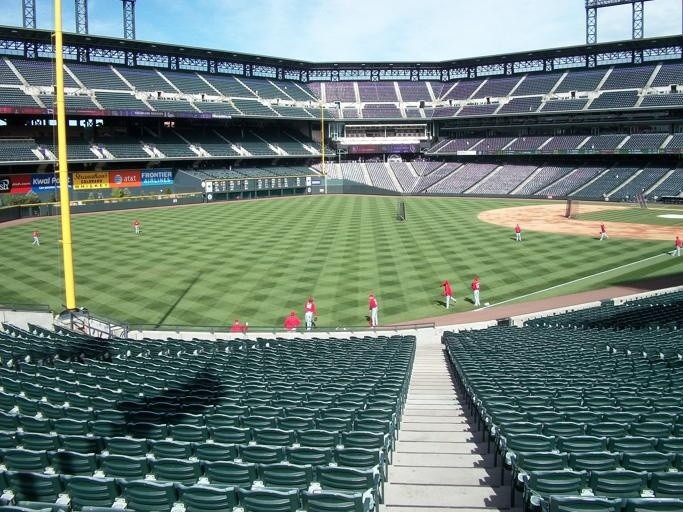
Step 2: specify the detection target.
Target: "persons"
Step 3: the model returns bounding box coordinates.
[440,280,457,310]
[229,320,248,332]
[600,223,608,240]
[672,237,683,257]
[472,276,481,307]
[32,229,40,246]
[88,191,103,200]
[368,294,379,329]
[285,311,301,330]
[515,224,521,242]
[133,218,141,235]
[304,298,320,331]
